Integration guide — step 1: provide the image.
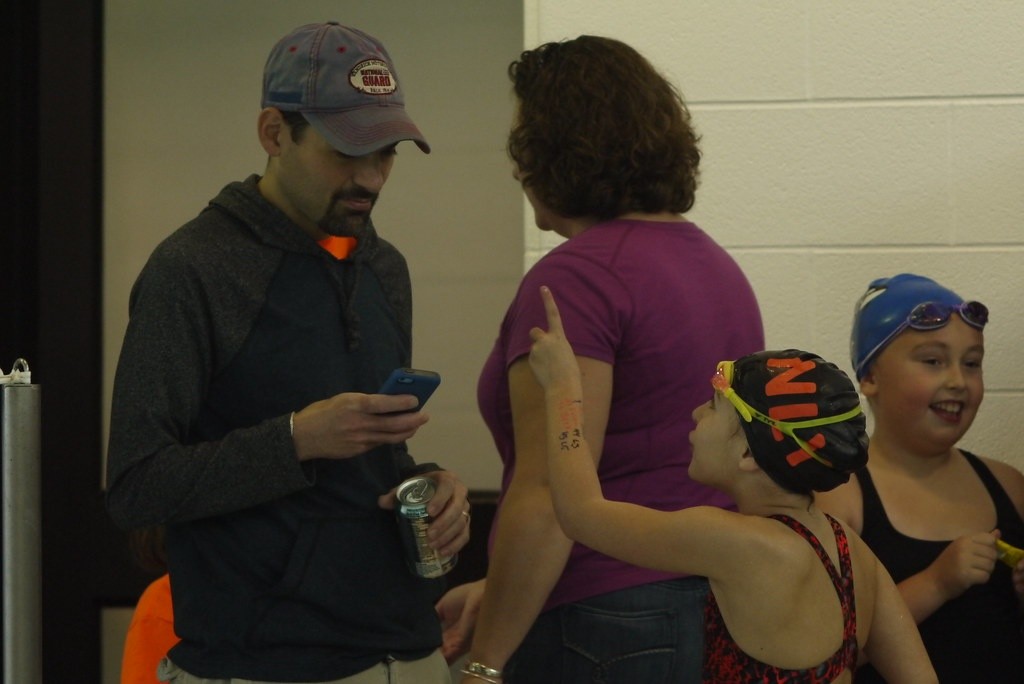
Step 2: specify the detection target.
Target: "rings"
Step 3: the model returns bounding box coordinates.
[462,511,469,522]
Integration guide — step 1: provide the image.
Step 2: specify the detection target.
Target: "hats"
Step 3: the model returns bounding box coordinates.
[849,270,967,377]
[262,21,433,155]
[729,348,871,495]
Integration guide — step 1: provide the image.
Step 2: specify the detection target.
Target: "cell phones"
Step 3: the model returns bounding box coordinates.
[374,368,441,417]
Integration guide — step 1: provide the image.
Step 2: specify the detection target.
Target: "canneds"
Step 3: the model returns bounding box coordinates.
[393,476,458,579]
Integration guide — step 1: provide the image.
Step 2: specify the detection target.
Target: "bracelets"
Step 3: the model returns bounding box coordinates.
[459,661,503,684]
[290,412,295,436]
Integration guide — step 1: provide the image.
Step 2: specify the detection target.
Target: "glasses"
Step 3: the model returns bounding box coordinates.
[712,359,791,436]
[859,300,988,375]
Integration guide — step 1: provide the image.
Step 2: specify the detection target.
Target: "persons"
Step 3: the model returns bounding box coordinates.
[826,273,1024,684]
[527,285,939,684]
[119,527,183,684]
[435,36,767,684]
[104,19,472,684]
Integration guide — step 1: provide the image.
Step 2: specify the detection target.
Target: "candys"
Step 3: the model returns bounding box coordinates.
[993,537,1024,568]
